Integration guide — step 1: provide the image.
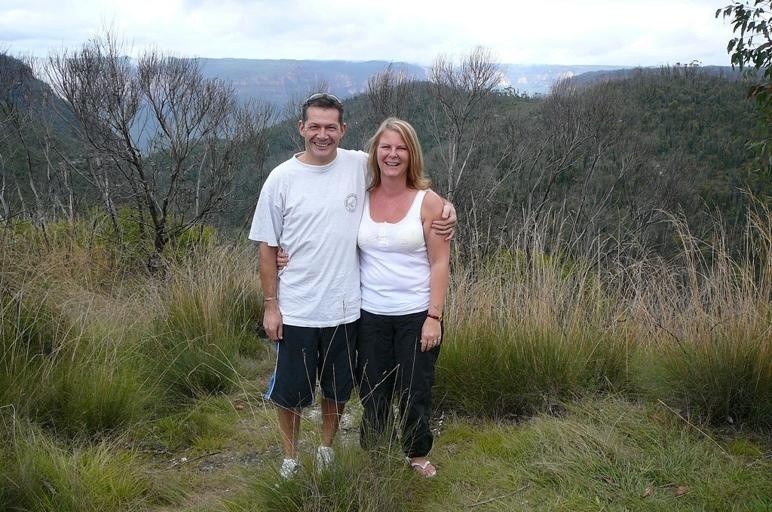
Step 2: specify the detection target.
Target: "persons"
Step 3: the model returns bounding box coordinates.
[274,116,451,480]
[247,92,460,478]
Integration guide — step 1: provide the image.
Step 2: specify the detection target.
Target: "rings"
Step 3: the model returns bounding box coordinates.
[450,228,452,232]
[433,341,437,344]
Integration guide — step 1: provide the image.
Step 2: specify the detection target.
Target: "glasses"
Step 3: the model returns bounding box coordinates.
[301,92,345,120]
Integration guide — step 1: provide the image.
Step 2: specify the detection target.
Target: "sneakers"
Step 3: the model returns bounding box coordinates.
[317,444,336,475]
[272,456,306,490]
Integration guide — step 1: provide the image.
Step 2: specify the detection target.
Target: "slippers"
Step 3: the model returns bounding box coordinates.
[406,455,437,478]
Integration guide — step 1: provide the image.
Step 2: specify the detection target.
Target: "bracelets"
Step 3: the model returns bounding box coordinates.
[262,296,277,303]
[426,313,443,321]
